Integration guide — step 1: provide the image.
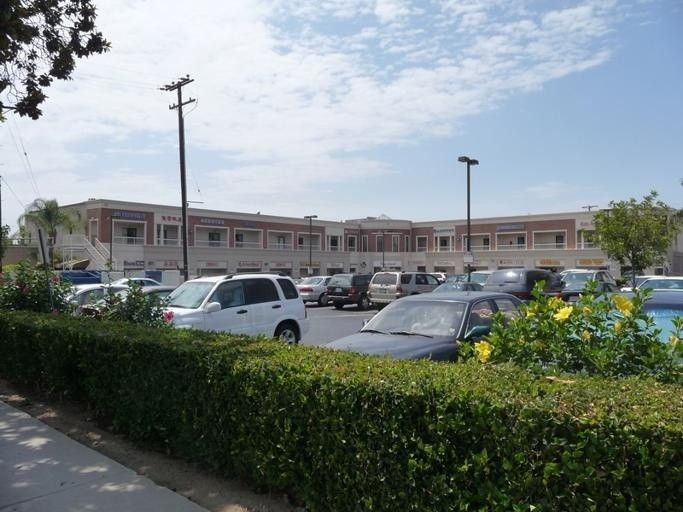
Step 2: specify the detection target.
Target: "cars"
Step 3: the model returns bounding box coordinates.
[528,290,683,382]
[48,272,175,317]
[637,275,683,290]
[316,288,528,366]
[620,275,655,291]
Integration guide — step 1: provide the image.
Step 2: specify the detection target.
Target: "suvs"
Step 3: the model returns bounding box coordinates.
[132,267,310,346]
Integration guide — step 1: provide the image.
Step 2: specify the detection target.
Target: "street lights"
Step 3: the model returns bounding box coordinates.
[581,205,598,211]
[456,155,479,271]
[378,229,389,271]
[303,214,317,268]
